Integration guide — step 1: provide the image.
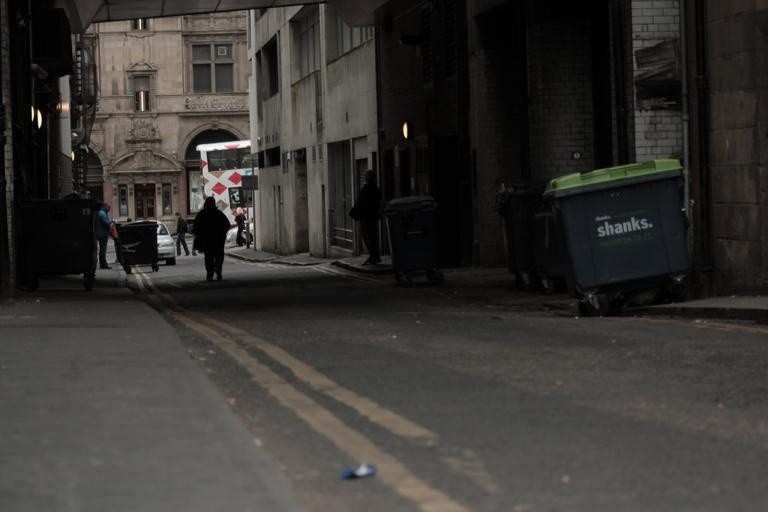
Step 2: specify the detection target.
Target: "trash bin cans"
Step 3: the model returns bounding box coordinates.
[543,159,691,317]
[384,196,445,287]
[119,221,159,274]
[15,197,101,292]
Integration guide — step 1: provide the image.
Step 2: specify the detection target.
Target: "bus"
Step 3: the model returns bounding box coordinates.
[195,140,255,226]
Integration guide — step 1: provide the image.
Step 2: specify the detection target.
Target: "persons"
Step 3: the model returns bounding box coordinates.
[193,197,231,280]
[348,170,383,265]
[235,207,246,246]
[94,203,115,269]
[175,213,190,256]
[192,234,203,256]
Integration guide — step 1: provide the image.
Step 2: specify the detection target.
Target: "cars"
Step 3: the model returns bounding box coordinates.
[227,219,256,244]
[137,220,176,265]
